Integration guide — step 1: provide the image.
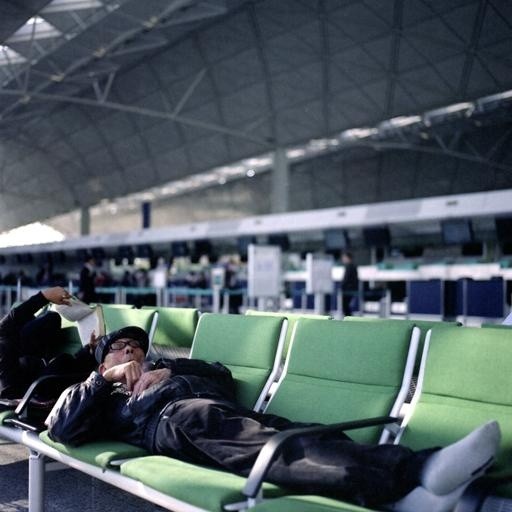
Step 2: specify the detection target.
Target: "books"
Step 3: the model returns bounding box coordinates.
[56,291,107,348]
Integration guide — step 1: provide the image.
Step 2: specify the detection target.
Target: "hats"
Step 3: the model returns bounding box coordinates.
[95,326,149,364]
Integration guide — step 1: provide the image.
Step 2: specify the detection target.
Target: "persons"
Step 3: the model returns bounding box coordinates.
[45,325,503,512]
[1,286,97,398]
[335,251,360,316]
[0,253,148,305]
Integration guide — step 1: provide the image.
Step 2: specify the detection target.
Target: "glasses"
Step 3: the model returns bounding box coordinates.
[109,340,141,350]
